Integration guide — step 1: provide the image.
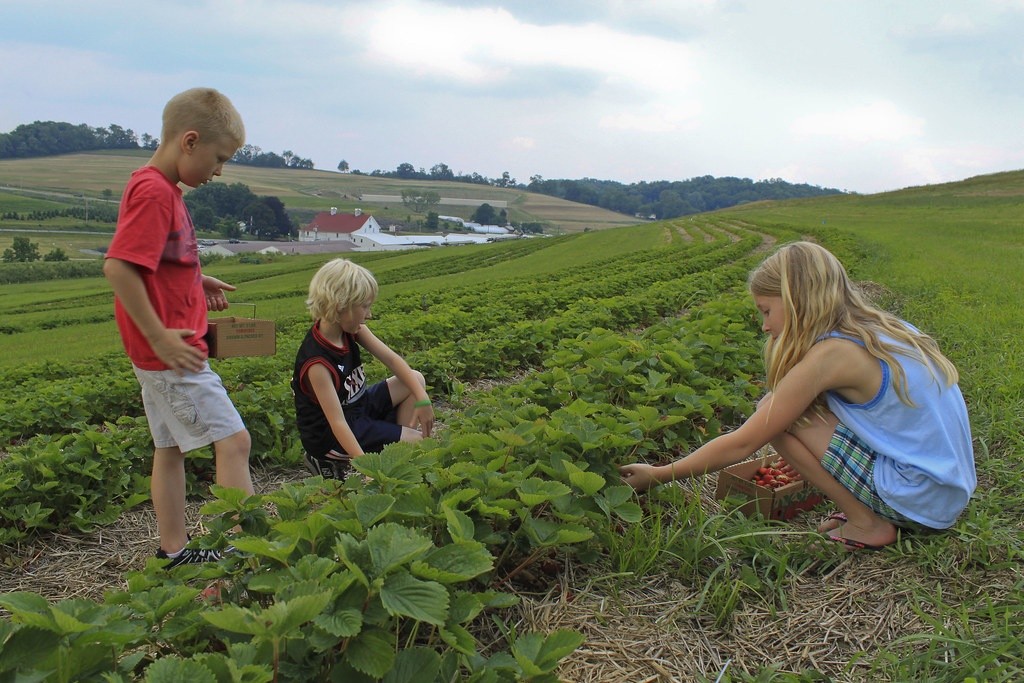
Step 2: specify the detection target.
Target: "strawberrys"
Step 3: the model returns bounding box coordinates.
[206,587,217,599]
[750,462,800,490]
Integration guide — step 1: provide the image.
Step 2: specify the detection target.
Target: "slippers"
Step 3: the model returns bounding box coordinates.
[817,510,847,532]
[806,531,887,553]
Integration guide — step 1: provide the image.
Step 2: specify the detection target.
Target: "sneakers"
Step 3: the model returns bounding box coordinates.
[223,544,243,556]
[156,533,222,571]
[304,451,362,484]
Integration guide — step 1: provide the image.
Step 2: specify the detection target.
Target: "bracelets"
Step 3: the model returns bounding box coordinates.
[415,401,432,407]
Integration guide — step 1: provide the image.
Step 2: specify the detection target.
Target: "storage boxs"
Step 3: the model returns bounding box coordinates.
[714,451,826,526]
[204,316,276,360]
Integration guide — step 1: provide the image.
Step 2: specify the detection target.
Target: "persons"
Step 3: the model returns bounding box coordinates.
[618,240,977,554]
[103,87,259,577]
[290,258,435,482]
